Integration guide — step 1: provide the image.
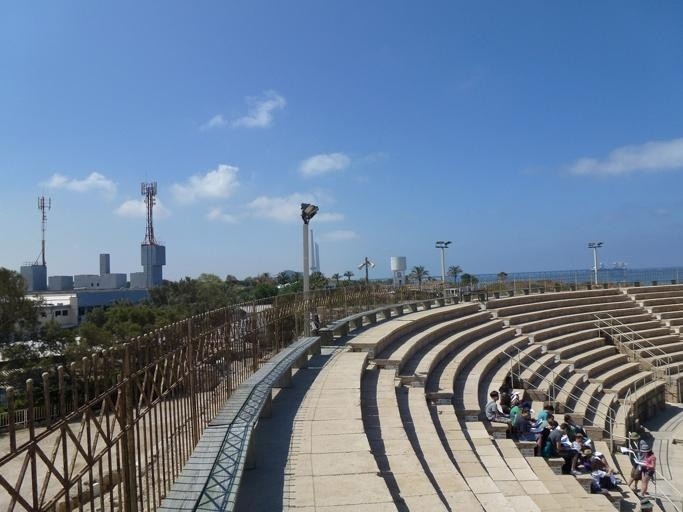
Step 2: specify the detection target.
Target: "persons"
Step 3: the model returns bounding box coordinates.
[620,431,656,499]
[484,391,621,502]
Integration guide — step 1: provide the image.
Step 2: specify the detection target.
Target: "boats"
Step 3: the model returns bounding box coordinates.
[611,261,628,270]
[590,262,608,271]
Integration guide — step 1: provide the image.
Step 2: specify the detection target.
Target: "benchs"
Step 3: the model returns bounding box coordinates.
[153,278,683,512]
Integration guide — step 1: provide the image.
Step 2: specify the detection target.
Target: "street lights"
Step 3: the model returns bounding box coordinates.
[434,239,452,304]
[299,201,319,335]
[358,256,374,286]
[587,240,602,285]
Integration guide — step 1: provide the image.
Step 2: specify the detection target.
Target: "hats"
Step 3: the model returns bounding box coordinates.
[630,432,640,439]
[640,444,652,452]
